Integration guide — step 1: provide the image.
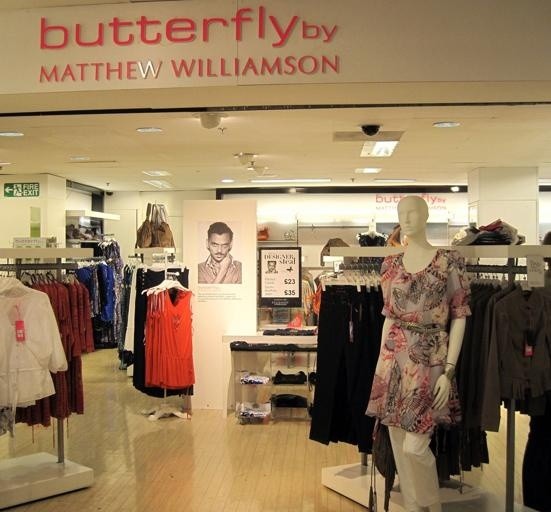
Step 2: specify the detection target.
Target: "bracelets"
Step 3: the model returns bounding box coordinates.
[442,370,452,381]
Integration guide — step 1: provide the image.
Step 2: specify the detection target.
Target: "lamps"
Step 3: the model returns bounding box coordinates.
[191,112,228,129]
[233,152,256,166]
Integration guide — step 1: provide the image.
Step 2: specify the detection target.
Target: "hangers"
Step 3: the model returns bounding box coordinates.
[127,256,192,298]
[0,231,118,288]
[318,260,536,294]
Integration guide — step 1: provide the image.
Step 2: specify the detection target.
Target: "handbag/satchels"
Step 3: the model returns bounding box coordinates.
[137,203,175,248]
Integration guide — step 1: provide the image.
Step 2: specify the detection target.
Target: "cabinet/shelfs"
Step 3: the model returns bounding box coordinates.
[224,334,320,425]
[67,209,121,243]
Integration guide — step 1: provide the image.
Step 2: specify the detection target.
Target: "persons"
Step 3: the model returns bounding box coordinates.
[362,194,476,512]
[264,260,277,274]
[198,220,241,284]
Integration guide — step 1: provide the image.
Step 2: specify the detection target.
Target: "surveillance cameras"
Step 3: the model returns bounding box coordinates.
[362,124,380,136]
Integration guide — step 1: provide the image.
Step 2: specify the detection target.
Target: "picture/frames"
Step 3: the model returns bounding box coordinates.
[259,247,302,301]
